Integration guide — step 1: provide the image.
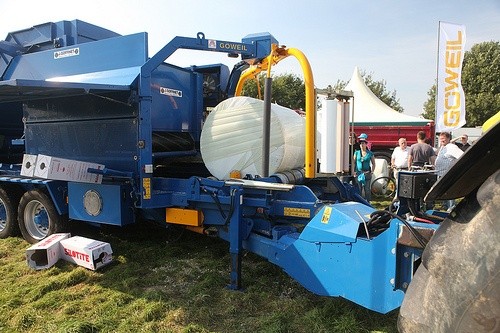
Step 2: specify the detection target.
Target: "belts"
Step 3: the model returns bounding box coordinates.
[401,168,408,170]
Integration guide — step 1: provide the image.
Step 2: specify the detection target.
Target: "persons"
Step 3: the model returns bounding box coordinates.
[391,130,472,213]
[349,132,375,202]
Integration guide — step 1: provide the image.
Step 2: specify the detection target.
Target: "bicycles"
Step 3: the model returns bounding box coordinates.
[370,167,398,200]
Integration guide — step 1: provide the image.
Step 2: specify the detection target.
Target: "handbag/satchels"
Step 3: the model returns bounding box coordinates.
[358,171,372,182]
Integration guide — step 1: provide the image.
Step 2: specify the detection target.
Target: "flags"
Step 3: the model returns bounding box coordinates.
[435,21,466,133]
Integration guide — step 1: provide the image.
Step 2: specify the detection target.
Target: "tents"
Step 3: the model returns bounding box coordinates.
[333,67,433,123]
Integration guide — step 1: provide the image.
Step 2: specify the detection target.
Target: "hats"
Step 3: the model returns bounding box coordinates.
[358,139,368,143]
[349,132,357,138]
[357,133,368,139]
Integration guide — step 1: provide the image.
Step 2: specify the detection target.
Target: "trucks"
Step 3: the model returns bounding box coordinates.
[349,122,436,163]
